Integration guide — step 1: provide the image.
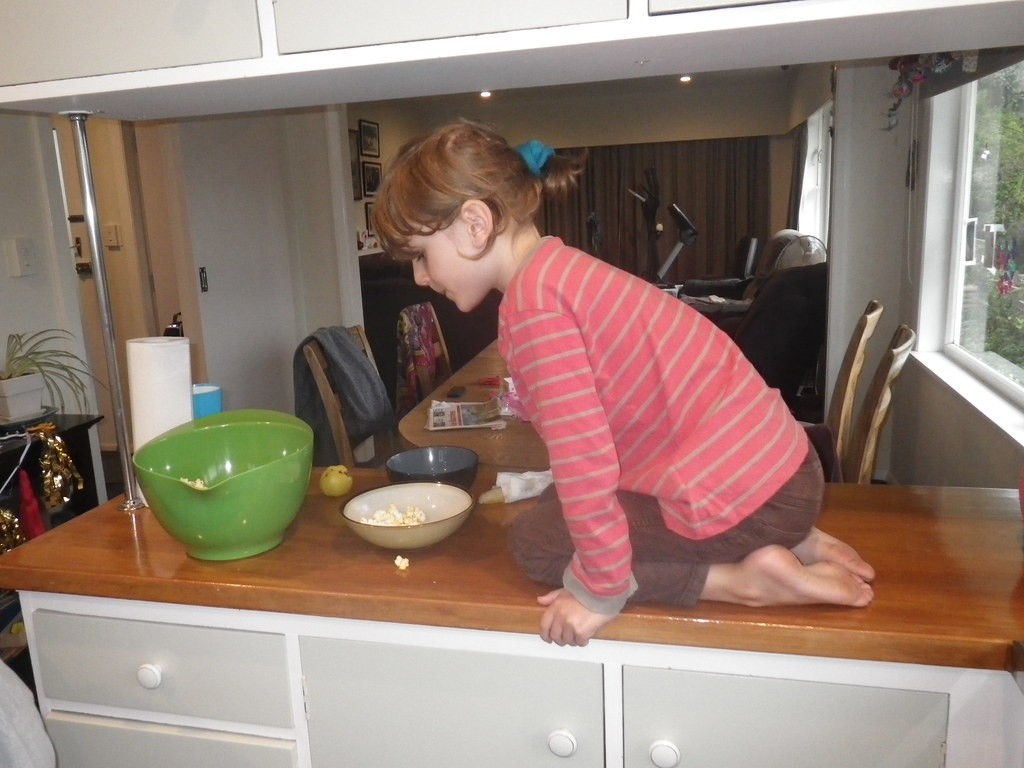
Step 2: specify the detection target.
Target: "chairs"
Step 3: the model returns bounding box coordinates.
[397,301,456,408]
[802,299,885,480]
[303,324,407,469]
[838,323,918,485]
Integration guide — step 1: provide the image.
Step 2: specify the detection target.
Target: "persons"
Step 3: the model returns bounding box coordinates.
[370,120,877,651]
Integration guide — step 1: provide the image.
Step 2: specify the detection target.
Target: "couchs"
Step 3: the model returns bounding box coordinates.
[677,229,804,341]
[715,235,826,414]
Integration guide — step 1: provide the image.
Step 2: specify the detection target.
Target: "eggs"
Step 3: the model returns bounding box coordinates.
[320,465,352,497]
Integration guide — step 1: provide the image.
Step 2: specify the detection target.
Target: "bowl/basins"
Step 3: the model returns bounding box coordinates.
[132,408,313,563]
[386,445,479,490]
[342,481,474,550]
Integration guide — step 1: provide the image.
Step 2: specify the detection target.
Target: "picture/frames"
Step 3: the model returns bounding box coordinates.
[365,201,376,237]
[362,161,385,198]
[346,128,364,201]
[359,119,380,158]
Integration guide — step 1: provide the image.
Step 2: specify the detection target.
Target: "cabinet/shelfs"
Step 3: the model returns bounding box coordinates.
[11,590,1023,767]
[0,0,267,86]
[0,413,105,611]
[273,0,807,55]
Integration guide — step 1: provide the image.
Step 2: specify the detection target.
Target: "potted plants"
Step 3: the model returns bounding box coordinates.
[0,327,106,424]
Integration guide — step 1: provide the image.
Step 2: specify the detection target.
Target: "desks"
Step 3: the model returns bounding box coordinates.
[398,323,555,473]
